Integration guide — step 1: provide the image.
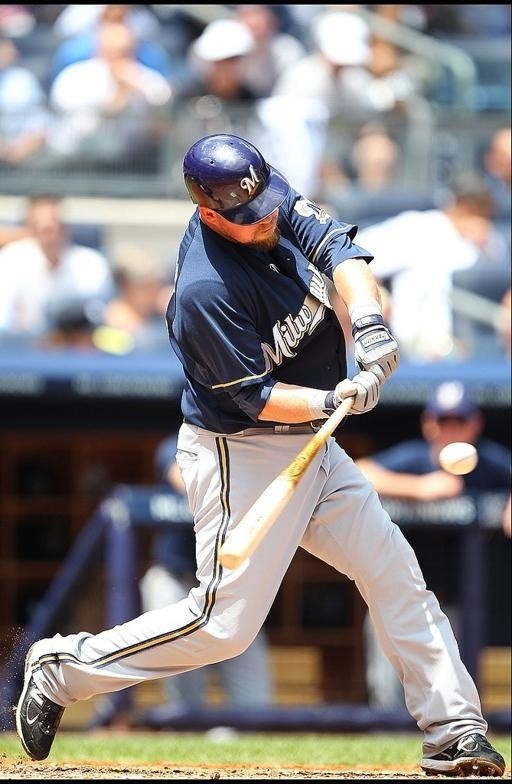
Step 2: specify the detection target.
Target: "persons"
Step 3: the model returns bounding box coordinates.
[348,375,512,707]
[15,131,506,775]
[134,432,274,711]
[1,2,511,360]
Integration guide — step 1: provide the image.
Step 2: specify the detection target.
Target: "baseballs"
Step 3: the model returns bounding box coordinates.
[442,441,478,476]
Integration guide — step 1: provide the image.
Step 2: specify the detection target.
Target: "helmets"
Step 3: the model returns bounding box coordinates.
[182,132,290,226]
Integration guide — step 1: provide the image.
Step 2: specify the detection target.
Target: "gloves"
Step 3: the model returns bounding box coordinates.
[306,371,379,422]
[350,295,399,380]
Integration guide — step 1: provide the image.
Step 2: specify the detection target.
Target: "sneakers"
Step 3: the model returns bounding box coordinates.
[15,636,65,759]
[420,733,505,775]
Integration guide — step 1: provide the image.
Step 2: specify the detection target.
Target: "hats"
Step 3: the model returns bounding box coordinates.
[430,380,476,423]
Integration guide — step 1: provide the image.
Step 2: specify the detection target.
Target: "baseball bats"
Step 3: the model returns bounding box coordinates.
[218,397,356,570]
